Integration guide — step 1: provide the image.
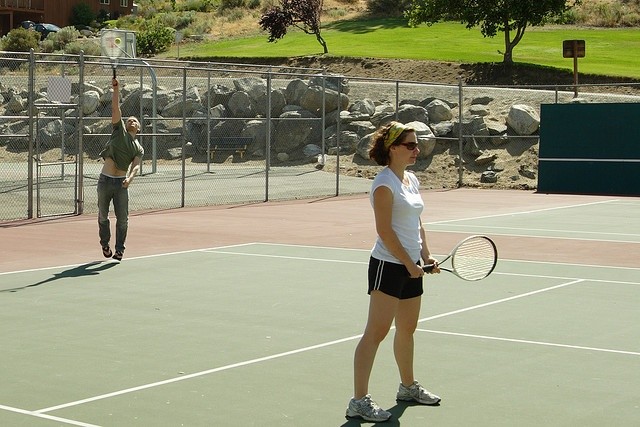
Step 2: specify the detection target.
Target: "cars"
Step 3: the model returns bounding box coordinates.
[22,19,63,41]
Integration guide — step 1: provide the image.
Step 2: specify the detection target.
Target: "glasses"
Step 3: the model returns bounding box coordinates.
[401,142,418,150]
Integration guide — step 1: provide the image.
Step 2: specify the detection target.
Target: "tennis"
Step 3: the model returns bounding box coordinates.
[114,38,121,45]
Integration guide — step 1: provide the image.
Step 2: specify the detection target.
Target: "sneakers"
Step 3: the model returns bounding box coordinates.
[115,249,124,259]
[102,244,112,258]
[396,380,441,404]
[345,394,392,422]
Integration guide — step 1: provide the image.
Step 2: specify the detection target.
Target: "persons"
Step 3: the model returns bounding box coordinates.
[96,77,146,262]
[344,119,444,421]
